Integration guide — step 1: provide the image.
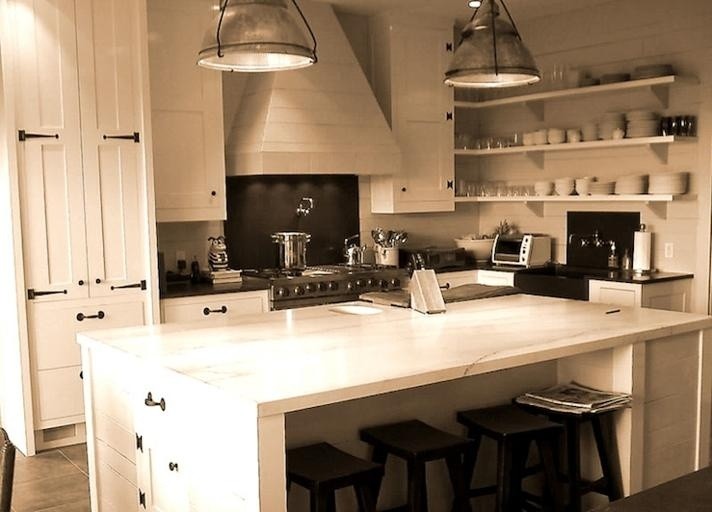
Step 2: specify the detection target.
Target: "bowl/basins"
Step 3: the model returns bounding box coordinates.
[453,237,495,264]
[544,61,585,90]
[533,176,594,196]
[522,129,581,146]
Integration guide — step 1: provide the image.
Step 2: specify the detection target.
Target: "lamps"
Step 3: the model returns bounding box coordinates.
[440,1,542,91]
[194,0,322,75]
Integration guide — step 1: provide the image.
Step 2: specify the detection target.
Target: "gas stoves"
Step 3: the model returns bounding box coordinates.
[242,265,407,301]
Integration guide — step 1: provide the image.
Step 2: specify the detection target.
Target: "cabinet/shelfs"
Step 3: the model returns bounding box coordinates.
[369,13,457,216]
[455,75,701,202]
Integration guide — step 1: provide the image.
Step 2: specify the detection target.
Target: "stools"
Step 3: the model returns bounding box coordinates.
[356,415,474,512]
[286,440,389,511]
[452,400,570,512]
[509,392,625,512]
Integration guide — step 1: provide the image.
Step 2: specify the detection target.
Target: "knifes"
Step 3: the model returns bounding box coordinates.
[405,252,424,278]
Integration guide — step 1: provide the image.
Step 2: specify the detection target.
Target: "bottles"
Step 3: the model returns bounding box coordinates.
[621,248,629,270]
[607,241,619,269]
[510,127,522,147]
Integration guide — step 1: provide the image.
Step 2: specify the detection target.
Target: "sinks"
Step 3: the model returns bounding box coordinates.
[330,303,386,316]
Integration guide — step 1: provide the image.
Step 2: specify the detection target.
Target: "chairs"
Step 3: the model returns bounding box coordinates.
[0,423,17,511]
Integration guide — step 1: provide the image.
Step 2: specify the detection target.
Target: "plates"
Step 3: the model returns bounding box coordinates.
[587,171,690,195]
[580,110,660,141]
[579,63,676,86]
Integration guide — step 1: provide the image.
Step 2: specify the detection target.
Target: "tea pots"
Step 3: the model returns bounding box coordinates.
[341,233,369,268]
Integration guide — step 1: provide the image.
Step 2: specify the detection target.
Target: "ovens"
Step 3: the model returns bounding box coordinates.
[513,273,589,301]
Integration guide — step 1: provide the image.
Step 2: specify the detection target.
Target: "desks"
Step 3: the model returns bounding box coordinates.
[73,292,711,512]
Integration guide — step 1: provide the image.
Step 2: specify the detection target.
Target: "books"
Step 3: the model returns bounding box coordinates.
[514,374,632,416]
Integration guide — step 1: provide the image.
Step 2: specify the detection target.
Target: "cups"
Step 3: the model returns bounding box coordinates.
[474,136,511,149]
[459,181,532,198]
[378,248,399,268]
[660,116,695,137]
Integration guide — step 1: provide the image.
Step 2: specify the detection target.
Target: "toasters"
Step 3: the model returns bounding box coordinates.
[490,231,552,268]
